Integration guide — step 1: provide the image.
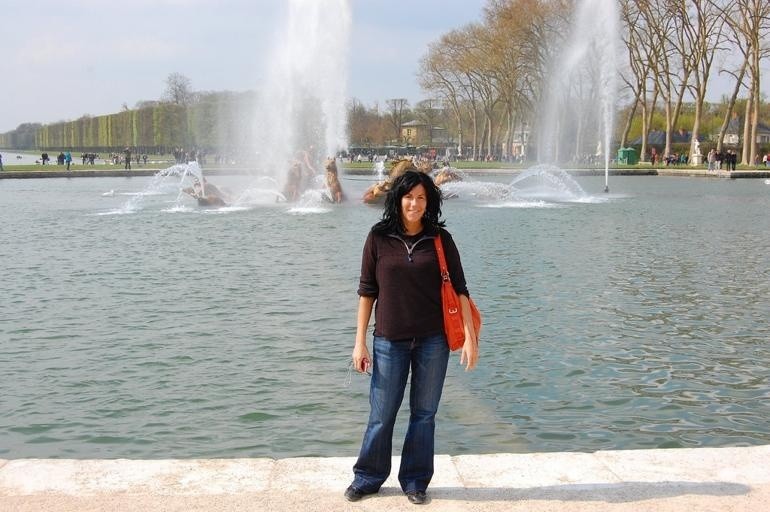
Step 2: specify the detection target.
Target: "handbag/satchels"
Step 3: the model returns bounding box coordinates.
[433,235,482,355]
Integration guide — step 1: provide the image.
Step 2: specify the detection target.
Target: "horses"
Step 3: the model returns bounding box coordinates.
[388,157,418,180]
[274,158,301,205]
[321,156,344,204]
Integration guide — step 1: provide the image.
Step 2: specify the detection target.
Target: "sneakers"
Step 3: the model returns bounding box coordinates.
[344,483,379,502]
[405,490,427,504]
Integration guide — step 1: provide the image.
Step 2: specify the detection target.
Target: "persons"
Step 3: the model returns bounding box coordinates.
[755,152,760,166]
[347,150,439,164]
[766,153,770,167]
[763,154,768,167]
[482,152,529,165]
[651,152,688,168]
[707,147,736,171]
[40,142,248,171]
[341,170,481,504]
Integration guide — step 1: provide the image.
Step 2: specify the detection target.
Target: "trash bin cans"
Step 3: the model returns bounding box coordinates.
[618,147,628,164]
[626,147,636,164]
